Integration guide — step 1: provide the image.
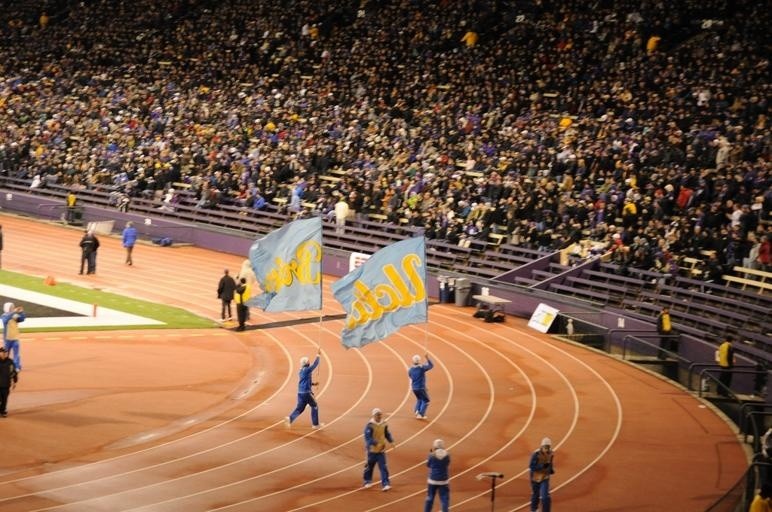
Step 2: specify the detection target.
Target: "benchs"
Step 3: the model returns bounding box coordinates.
[2,171,421,263]
[421,213,772,373]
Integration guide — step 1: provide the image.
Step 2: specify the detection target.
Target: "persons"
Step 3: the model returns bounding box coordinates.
[0,302,25,373]
[407,351,434,420]
[423,438,450,512]
[655,307,673,359]
[286,349,324,430]
[530,437,555,511]
[362,407,394,492]
[750,482,772,512]
[717,336,736,397]
[0,347,17,418]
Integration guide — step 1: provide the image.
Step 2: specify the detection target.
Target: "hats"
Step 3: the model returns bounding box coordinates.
[301,357,308,367]
[413,355,421,363]
[434,440,444,448]
[373,408,381,415]
[542,438,551,446]
[0,347,8,352]
[4,301,15,312]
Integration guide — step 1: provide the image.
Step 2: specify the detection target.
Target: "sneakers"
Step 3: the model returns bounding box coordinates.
[312,423,324,429]
[365,483,372,488]
[383,484,391,491]
[417,414,427,419]
[286,416,291,428]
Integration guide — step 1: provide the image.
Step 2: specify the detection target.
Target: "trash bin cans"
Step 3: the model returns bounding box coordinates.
[437,275,472,306]
